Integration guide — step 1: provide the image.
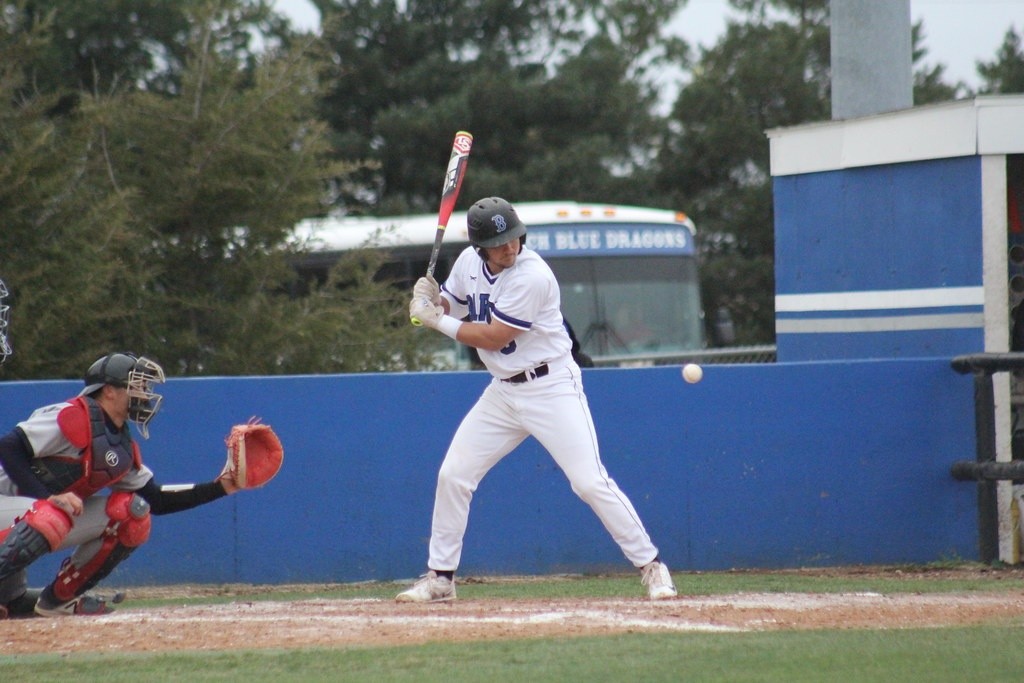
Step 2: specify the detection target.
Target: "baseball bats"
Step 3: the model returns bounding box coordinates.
[409,130,473,326]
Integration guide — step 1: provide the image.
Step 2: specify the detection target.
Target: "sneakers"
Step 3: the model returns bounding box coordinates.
[0,589,43,618]
[640,561,678,600]
[395,571,456,603]
[34,583,115,617]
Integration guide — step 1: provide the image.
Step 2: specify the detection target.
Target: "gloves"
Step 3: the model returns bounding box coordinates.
[409,298,439,328]
[413,273,442,305]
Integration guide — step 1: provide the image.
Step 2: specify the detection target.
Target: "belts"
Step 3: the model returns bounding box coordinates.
[500,364,549,383]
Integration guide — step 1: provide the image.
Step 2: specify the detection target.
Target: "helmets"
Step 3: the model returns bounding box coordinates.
[467,196,527,261]
[76,352,157,399]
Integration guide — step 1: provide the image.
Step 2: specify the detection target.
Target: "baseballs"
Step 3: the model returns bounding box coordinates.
[683,364,702,383]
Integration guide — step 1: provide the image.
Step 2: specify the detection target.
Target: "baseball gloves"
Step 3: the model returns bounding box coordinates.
[215,424,284,490]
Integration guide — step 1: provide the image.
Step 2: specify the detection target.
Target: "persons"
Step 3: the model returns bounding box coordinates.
[395,197,676,601]
[0,352,285,618]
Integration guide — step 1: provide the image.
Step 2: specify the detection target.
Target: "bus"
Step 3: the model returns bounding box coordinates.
[144,200,706,371]
